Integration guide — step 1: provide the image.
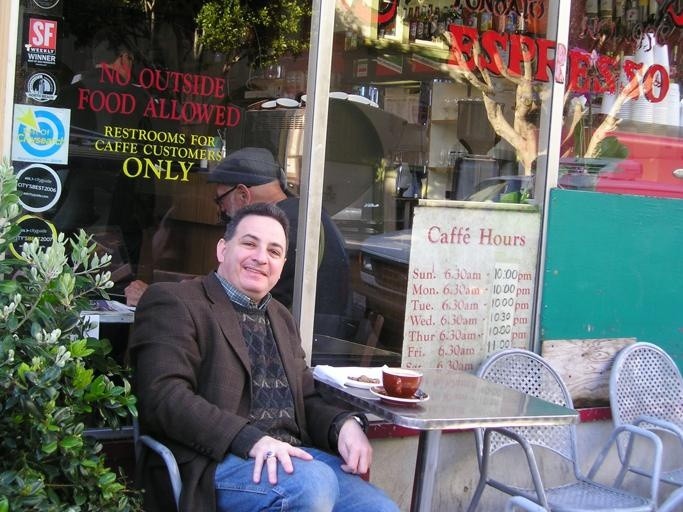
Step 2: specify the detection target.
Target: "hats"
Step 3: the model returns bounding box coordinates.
[204,147,281,188]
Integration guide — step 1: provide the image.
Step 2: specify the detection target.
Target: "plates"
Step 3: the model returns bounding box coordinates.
[371,385,430,405]
[338,364,381,393]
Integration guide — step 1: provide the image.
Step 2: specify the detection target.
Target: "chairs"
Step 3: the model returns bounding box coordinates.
[605,336,683,511]
[466,349,670,512]
[126,389,371,512]
[346,306,384,366]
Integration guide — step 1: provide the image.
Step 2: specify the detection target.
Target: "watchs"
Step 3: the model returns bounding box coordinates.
[352,415,364,430]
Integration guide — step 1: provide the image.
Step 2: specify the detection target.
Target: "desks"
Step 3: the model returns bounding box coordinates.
[69,300,136,376]
[307,363,581,510]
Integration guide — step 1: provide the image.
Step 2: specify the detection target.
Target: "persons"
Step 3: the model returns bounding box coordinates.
[129,204,397,512]
[125,147,352,334]
[20,16,166,281]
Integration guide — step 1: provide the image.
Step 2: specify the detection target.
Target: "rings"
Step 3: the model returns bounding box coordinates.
[264,450,275,460]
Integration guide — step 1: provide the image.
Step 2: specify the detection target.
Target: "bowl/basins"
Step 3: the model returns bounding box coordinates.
[381,366,425,396]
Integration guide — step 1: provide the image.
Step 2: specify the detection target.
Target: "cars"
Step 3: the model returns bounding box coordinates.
[357,169,603,336]
